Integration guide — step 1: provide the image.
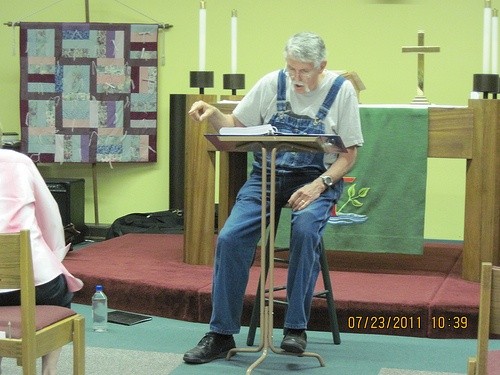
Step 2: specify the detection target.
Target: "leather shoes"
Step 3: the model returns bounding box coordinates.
[183,334,236,363]
[280,329,307,354]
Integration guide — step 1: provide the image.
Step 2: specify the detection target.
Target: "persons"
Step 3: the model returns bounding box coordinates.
[0,151,84,375]
[182,32,364,363]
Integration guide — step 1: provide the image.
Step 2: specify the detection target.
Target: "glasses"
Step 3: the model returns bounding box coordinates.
[283,65,316,78]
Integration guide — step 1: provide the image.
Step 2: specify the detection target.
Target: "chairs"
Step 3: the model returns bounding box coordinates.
[0,230,86,375]
[468,262,500,375]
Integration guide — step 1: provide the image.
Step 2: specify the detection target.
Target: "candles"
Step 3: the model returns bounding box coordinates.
[491,8,500,75]
[231,9,237,74]
[198,0,205,71]
[483,0,492,74]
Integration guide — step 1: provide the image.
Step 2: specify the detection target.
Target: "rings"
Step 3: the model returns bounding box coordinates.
[302,200,304,203]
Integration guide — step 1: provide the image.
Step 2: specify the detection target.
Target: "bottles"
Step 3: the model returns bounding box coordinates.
[92,285,108,332]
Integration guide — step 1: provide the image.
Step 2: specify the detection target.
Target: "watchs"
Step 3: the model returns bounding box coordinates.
[320,175,333,188]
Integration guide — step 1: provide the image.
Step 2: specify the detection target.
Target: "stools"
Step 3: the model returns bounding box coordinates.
[246,203,342,345]
